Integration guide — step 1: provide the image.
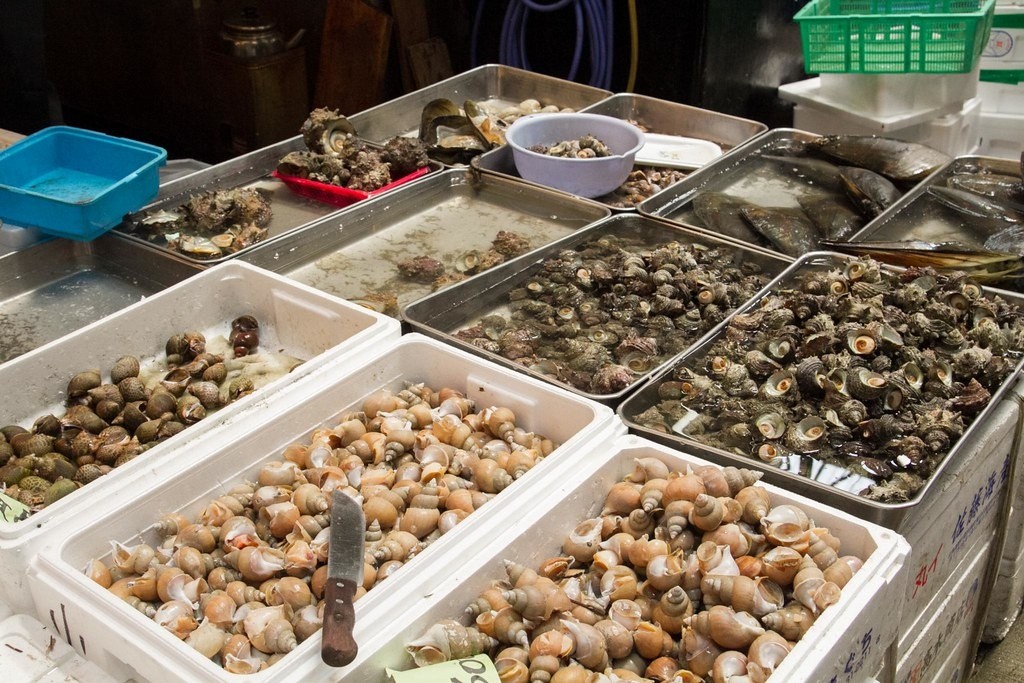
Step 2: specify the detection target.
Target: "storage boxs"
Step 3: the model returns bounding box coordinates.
[1,0,1024,683]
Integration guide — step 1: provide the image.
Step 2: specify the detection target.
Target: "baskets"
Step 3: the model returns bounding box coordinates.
[791,0,997,75]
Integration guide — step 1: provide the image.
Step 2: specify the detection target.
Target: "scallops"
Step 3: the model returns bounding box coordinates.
[121,105,430,261]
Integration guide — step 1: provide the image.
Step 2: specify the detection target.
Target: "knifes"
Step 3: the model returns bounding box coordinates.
[321,487,366,666]
